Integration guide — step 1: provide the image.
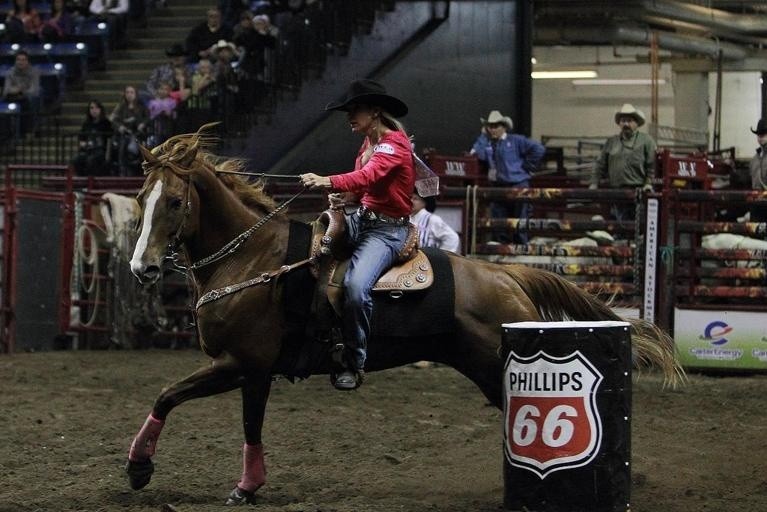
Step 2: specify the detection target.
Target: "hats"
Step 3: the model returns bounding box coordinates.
[615,103,645,127]
[165,42,192,57]
[750,119,767,135]
[479,110,513,132]
[413,186,435,213]
[325,80,409,119]
[210,39,237,53]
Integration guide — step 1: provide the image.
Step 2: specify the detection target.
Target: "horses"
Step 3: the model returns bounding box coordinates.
[123,122,688,505]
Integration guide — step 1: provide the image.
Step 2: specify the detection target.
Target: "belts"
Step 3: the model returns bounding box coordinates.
[355,204,411,227]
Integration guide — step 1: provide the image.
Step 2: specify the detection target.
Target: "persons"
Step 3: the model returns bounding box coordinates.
[212,39,241,93]
[588,104,658,303]
[0,0,128,42]
[233,10,254,40]
[2,50,40,98]
[145,43,197,93]
[408,188,460,368]
[748,119,767,192]
[231,15,280,80]
[186,10,230,62]
[192,59,217,110]
[300,80,415,391]
[112,85,151,177]
[145,85,177,120]
[71,99,115,176]
[468,111,545,245]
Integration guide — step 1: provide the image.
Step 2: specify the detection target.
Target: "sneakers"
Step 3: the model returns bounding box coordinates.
[334,369,357,388]
